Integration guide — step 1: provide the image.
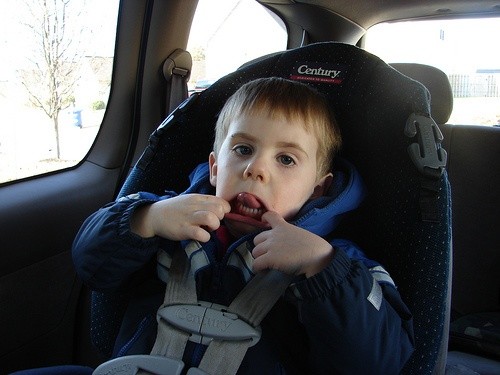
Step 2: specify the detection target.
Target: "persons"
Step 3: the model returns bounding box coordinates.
[71,78,417,375]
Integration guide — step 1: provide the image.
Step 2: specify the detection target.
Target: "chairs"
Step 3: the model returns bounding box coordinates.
[88,39,453,375]
[389,65,454,185]
